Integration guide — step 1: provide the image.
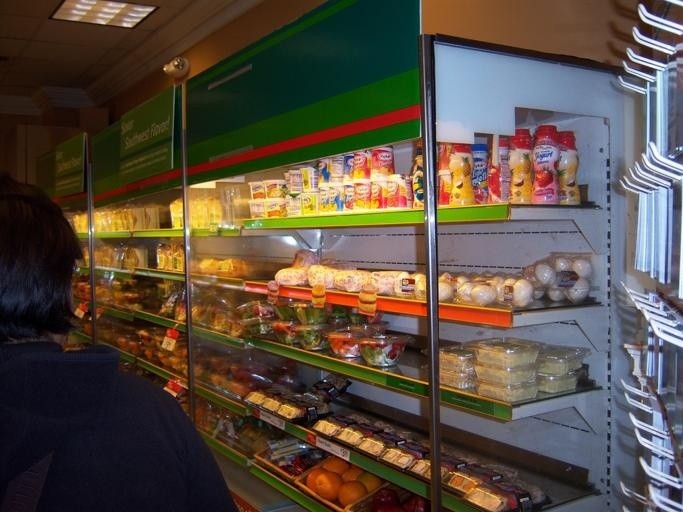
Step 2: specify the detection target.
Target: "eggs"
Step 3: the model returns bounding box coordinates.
[393,255,593,307]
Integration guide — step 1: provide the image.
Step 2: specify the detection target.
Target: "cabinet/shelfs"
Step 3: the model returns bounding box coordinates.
[35,131,91,354]
[87,94,193,428]
[181,0,643,512]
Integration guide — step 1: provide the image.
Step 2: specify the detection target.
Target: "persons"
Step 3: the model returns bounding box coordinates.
[1,179,238,512]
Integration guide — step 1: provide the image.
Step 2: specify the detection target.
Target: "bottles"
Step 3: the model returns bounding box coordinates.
[412,124,584,207]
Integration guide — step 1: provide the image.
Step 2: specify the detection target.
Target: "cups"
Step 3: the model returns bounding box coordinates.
[247,143,412,218]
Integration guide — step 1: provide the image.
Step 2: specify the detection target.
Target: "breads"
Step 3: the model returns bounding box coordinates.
[439,341,583,403]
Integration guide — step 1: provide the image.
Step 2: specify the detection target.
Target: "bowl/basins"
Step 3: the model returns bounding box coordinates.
[234,300,407,368]
